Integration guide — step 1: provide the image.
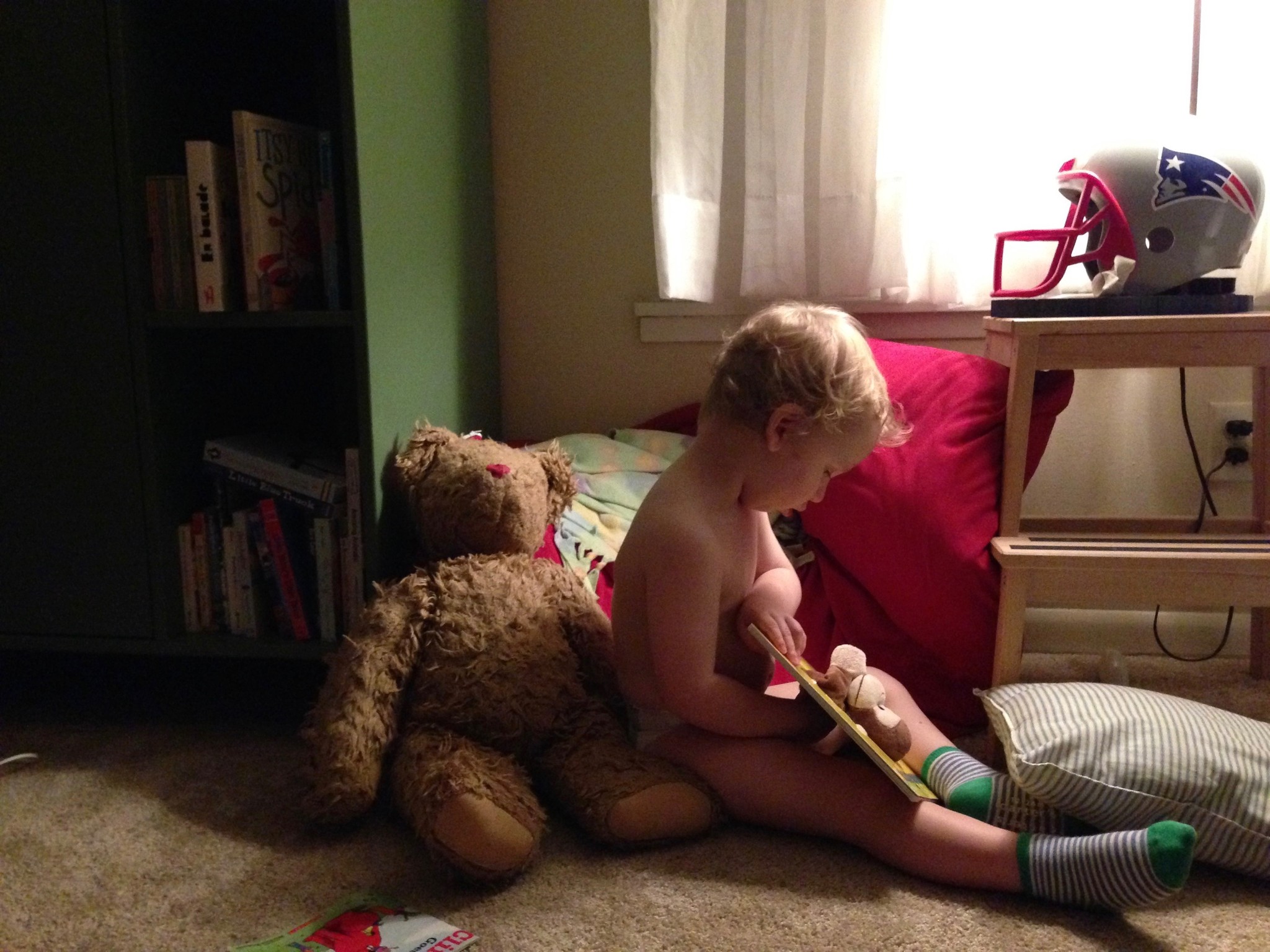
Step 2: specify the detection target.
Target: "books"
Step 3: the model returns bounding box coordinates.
[176,430,368,637]
[146,108,335,323]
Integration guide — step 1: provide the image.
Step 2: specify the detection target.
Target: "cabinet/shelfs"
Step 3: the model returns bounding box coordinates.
[0,0,383,662]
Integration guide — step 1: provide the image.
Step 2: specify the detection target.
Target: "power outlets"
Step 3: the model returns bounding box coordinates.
[1206,400,1253,483]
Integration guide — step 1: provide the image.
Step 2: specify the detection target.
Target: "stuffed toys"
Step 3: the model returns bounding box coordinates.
[327,422,711,865]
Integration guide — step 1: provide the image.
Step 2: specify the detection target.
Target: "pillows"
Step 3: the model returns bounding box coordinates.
[973,681,1269,876]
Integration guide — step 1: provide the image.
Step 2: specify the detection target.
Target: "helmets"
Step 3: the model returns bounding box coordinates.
[984,135,1265,296]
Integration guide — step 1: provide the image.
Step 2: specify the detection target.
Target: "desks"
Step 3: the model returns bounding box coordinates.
[979,310,1270,763]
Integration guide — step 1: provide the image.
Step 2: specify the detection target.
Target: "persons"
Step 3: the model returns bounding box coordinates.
[606,297,1205,909]
